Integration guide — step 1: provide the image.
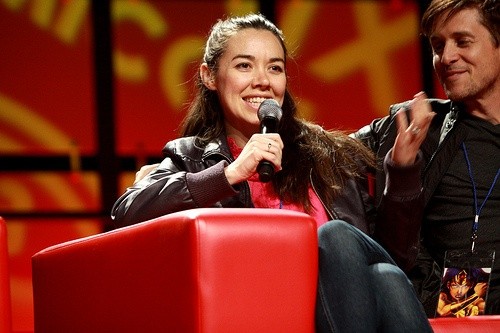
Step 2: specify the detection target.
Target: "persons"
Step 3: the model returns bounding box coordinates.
[111,14,437,333]
[132,0,500,319]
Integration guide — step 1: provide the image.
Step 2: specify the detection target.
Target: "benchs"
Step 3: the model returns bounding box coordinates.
[32,208,500,333]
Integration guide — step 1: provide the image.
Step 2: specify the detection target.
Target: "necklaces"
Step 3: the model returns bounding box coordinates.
[279,171,282,209]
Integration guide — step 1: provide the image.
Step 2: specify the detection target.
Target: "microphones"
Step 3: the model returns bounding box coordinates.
[257,99,282,181]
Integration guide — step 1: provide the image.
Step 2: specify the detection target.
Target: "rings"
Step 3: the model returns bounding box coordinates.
[408,122,422,134]
[268,143,272,152]
